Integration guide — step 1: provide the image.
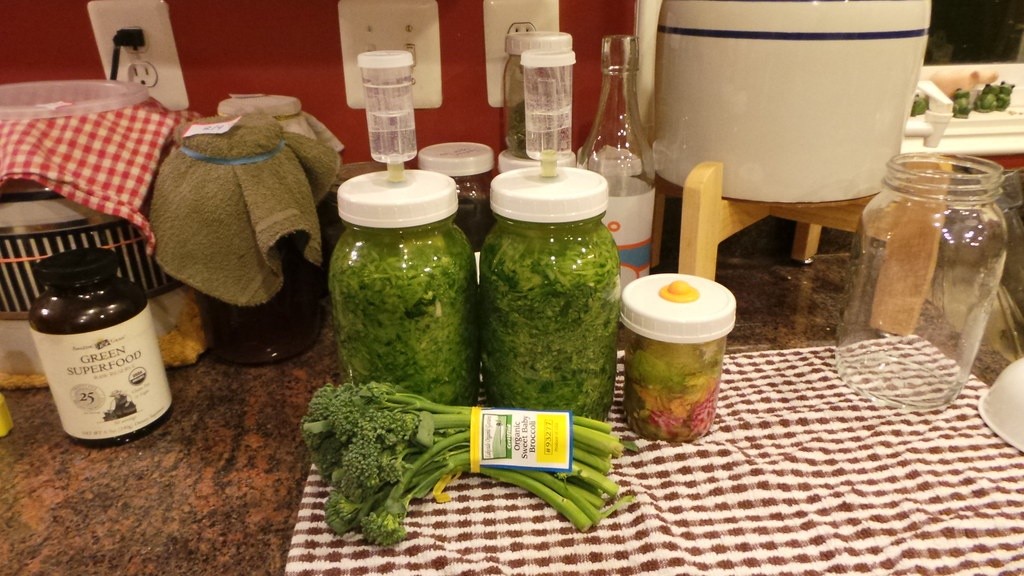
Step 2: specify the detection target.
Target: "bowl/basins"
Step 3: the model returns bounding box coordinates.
[978,355,1023,456]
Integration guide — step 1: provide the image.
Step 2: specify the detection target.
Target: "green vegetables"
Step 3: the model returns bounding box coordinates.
[297,380,624,545]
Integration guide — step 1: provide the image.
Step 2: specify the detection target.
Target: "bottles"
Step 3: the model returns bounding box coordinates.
[503,32,531,157]
[576,35,657,329]
[837,152,1006,409]
[418,143,497,263]
[28,246,174,451]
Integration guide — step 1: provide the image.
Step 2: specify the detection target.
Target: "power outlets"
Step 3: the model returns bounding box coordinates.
[87,0,192,112]
[339,0,441,109]
[484,0,560,107]
